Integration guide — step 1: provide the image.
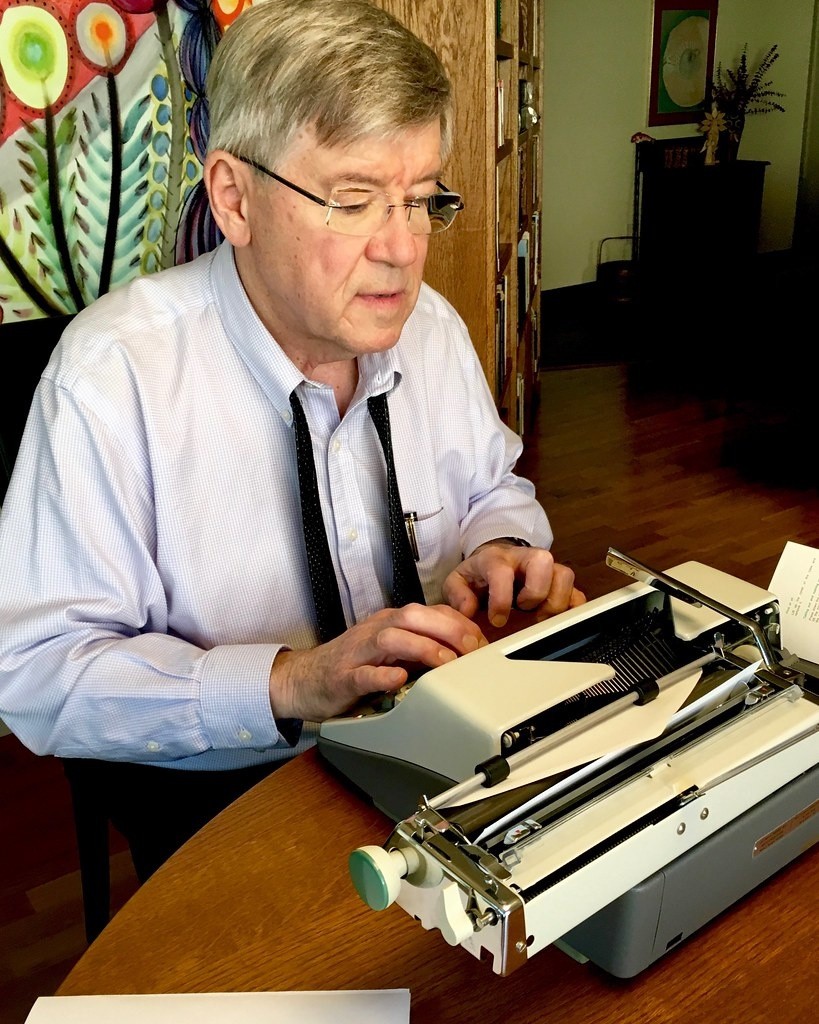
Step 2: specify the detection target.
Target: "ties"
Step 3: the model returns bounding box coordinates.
[289,389,426,647]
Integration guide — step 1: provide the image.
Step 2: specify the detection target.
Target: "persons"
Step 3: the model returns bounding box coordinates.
[0,0,588,871]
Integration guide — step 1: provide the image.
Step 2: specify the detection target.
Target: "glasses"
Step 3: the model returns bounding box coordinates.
[227,148,466,237]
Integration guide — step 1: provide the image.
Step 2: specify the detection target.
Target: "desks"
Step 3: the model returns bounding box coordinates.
[44,713,818,1023]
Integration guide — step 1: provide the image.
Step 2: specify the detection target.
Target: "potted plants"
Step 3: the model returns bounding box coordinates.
[695,35,789,158]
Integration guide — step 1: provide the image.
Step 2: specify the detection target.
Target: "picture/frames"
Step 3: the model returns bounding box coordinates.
[646,3,719,127]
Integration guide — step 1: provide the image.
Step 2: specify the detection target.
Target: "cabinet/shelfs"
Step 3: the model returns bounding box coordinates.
[351,1,541,494]
